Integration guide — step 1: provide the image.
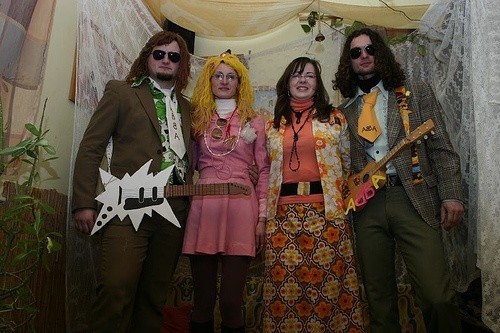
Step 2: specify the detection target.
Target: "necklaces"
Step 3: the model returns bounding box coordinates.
[285,101,319,171]
[200,100,245,158]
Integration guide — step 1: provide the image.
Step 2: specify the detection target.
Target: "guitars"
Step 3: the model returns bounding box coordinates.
[90,159,252,237]
[343,119,436,213]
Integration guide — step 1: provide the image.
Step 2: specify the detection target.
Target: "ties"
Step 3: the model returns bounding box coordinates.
[147,78,187,160]
[358,88,382,142]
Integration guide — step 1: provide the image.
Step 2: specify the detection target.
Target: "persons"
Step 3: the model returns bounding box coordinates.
[332,27,466,333]
[181,53,270,333]
[261,57,374,332]
[70,31,259,333]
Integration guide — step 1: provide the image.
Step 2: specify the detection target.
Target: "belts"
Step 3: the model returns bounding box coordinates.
[280,181,323,196]
[387,176,402,186]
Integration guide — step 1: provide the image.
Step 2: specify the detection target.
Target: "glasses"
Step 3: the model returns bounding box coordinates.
[349,44,375,59]
[212,74,239,80]
[150,51,181,63]
[290,73,317,79]
